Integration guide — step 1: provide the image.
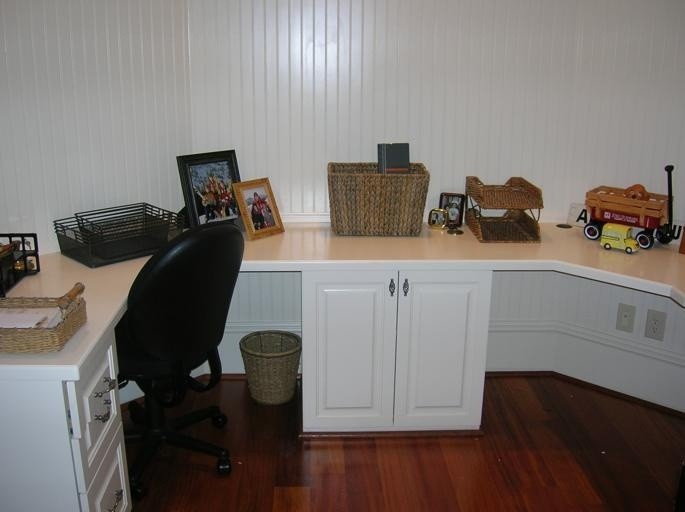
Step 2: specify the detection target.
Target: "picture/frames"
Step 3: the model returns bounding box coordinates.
[440,192,466,227]
[176,150,246,232]
[429,207,449,230]
[232,177,286,241]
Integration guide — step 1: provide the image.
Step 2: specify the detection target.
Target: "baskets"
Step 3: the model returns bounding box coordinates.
[327,162,430,236]
[0,297,87,354]
[53,202,181,268]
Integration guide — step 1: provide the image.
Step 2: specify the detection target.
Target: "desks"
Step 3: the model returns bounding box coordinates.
[1,221,685,512]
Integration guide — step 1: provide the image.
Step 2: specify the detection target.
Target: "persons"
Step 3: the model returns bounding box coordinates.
[432,196,459,225]
[250,191,274,230]
[199,175,241,224]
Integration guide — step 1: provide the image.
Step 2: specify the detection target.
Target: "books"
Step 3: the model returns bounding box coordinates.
[377,141,410,173]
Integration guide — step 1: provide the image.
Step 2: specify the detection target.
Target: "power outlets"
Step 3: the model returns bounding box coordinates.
[616,301,636,334]
[644,309,668,342]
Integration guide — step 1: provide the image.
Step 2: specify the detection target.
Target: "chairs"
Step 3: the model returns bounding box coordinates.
[119,224,244,500]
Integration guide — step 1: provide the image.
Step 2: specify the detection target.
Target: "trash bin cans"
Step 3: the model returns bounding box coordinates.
[240,330,302,406]
